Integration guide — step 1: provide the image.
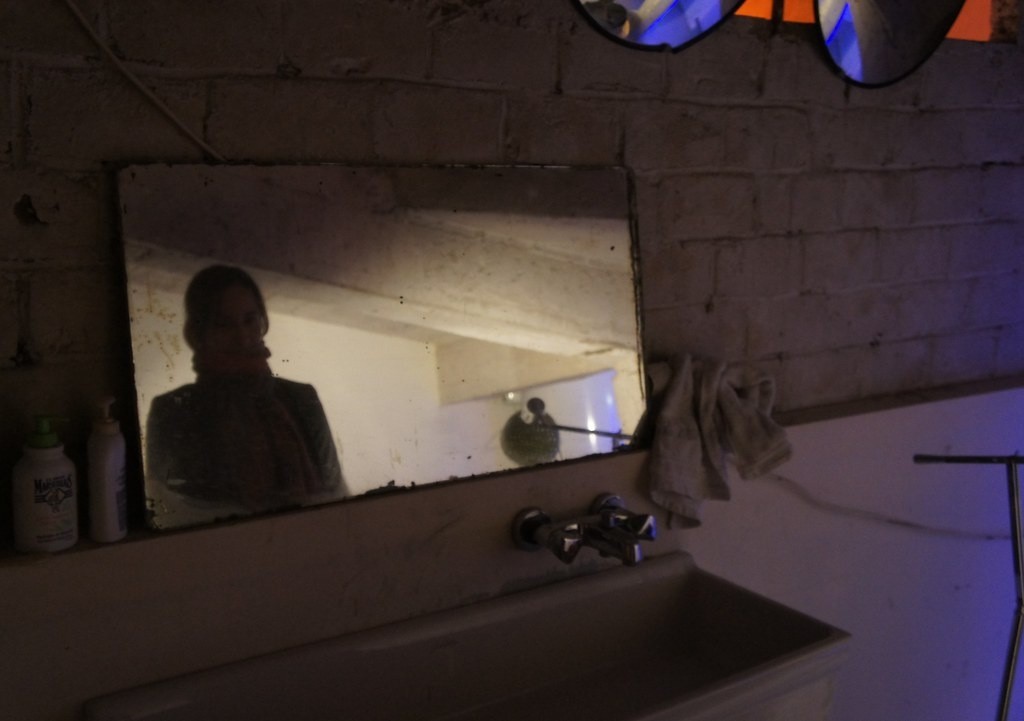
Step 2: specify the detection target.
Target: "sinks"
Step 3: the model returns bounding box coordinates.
[83,551,850,721]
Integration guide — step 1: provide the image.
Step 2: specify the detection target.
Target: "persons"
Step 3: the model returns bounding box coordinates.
[145,265,351,525]
[583,0,630,43]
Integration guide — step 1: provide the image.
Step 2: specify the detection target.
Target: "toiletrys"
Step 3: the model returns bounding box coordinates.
[10,412,79,557]
[87,391,126,544]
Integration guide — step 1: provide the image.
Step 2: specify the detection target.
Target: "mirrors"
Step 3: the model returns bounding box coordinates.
[111,158,653,534]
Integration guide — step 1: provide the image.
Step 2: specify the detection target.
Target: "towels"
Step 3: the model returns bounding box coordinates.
[648,353,796,532]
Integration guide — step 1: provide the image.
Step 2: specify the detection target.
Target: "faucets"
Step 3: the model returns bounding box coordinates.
[582,507,645,568]
